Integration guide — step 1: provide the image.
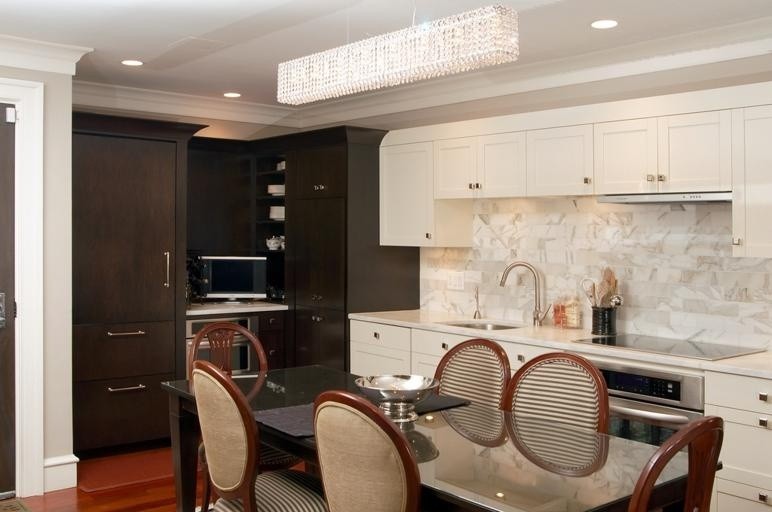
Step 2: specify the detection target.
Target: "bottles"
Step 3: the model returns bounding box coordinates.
[551,293,580,328]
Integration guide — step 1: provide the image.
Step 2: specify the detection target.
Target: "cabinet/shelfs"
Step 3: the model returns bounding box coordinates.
[703,366,772,511]
[351,319,411,377]
[187,144,253,256]
[525,119,594,201]
[253,153,290,253]
[380,139,470,251]
[433,127,525,202]
[410,328,484,383]
[73,111,213,458]
[293,126,424,372]
[724,104,772,261]
[594,106,732,194]
[487,338,565,384]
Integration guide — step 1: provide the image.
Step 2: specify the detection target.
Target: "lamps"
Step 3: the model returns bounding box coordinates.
[270,3,520,109]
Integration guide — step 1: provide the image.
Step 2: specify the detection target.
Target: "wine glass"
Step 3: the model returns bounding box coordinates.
[382,424,441,466]
[354,373,440,423]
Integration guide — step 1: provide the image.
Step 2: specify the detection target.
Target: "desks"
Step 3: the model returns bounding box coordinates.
[184,297,290,317]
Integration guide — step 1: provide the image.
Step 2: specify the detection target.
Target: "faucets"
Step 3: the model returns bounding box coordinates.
[498,260,542,325]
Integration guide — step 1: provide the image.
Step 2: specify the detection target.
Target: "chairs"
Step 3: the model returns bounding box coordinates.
[430,339,509,414]
[190,360,332,512]
[190,319,311,512]
[630,414,725,512]
[312,389,420,512]
[503,413,608,479]
[438,396,509,449]
[500,350,611,439]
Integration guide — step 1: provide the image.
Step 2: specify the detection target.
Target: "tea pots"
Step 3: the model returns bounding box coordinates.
[264,235,285,251]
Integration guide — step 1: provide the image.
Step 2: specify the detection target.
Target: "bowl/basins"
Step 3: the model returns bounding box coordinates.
[267,183,286,197]
[269,204,286,222]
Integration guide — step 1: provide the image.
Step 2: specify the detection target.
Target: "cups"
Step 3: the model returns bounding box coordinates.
[591,304,619,337]
[276,161,285,172]
[591,338,618,347]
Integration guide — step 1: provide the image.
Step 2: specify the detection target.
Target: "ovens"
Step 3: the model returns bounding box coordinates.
[566,354,705,454]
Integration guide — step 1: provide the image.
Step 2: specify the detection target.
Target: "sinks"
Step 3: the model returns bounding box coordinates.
[439,320,522,333]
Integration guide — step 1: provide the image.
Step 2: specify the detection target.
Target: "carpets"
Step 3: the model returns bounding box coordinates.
[0,495,35,511]
